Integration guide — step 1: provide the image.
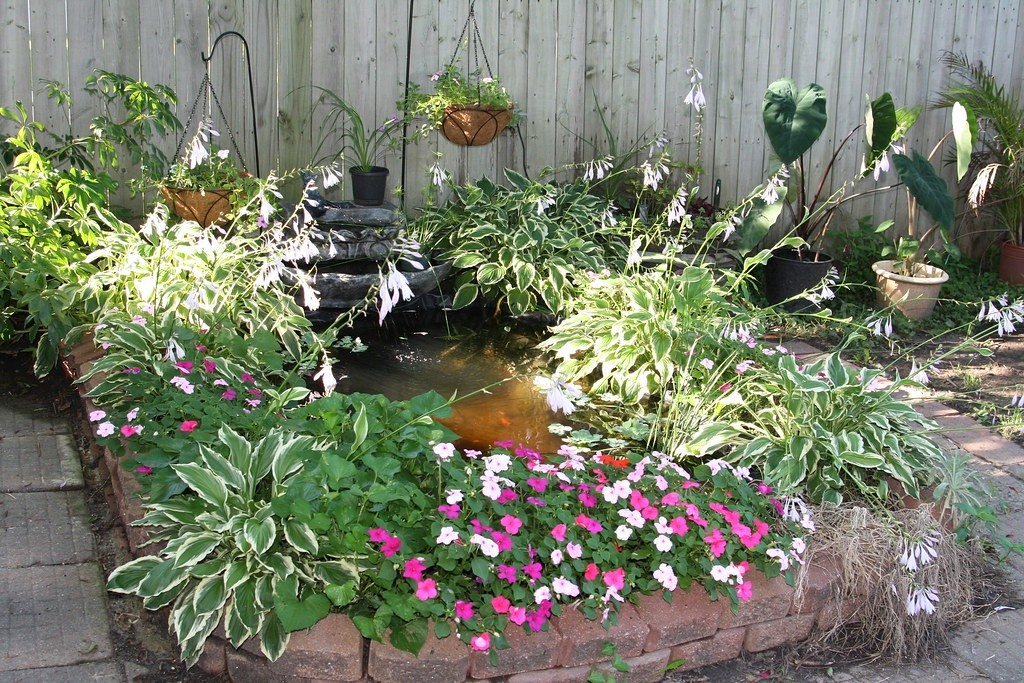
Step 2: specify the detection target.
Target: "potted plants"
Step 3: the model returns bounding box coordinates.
[128,124,261,229]
[754,83,898,315]
[870,98,980,319]
[285,85,414,206]
[926,49,1024,290]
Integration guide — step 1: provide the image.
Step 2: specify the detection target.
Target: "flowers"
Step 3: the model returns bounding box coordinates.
[399,66,523,147]
